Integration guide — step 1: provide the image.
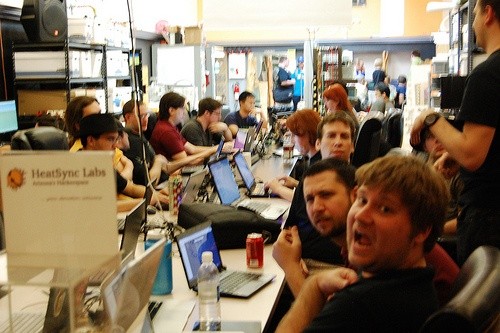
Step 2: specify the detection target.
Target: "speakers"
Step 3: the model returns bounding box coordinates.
[20,0,66,45]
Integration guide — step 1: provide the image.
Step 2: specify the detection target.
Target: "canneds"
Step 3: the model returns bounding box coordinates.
[168,174,182,215]
[246,233,264,268]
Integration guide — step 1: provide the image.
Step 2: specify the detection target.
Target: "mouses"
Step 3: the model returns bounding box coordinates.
[147,205,157,215]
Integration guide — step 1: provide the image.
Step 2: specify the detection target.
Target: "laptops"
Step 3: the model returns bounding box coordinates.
[0,119,291,333]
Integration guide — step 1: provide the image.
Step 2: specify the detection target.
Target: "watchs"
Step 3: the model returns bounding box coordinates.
[422,112,442,129]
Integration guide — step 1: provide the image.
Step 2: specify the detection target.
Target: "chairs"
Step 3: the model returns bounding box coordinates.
[351,109,404,169]
[419,246,500,333]
[10,127,69,151]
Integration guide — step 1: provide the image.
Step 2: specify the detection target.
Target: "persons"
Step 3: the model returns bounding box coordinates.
[34,92,259,214]
[264,56,461,333]
[410,0,500,260]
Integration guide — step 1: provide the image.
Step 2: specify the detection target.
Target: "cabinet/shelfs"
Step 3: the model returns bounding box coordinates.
[10,39,131,130]
[431,0,486,108]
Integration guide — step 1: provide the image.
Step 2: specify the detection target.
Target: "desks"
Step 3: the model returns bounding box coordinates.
[0,146,299,333]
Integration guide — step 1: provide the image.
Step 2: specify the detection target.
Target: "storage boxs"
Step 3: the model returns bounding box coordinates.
[15,19,133,119]
[462,23,482,49]
[460,53,486,76]
[185,26,202,44]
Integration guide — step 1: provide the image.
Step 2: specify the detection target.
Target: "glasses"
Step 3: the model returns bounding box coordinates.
[130,113,150,120]
[101,135,121,143]
[213,110,222,117]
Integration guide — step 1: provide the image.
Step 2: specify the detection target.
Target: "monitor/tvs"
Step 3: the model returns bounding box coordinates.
[0,100,19,135]
